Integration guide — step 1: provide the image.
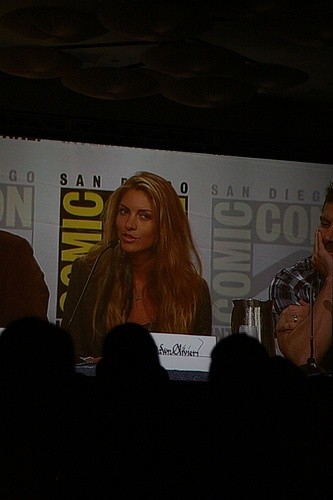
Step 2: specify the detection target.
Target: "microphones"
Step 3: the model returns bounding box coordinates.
[66,239,118,330]
[299,272,328,376]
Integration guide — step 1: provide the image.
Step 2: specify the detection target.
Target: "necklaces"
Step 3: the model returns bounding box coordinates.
[131,281,145,303]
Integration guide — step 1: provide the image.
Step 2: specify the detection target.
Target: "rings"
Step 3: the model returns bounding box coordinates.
[293,315,298,323]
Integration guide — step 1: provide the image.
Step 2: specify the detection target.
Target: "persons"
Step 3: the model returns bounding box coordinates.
[0,314,333,500]
[61,170,212,368]
[268,181,333,368]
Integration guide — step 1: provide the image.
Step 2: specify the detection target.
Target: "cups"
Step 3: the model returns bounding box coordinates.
[230,298,277,358]
